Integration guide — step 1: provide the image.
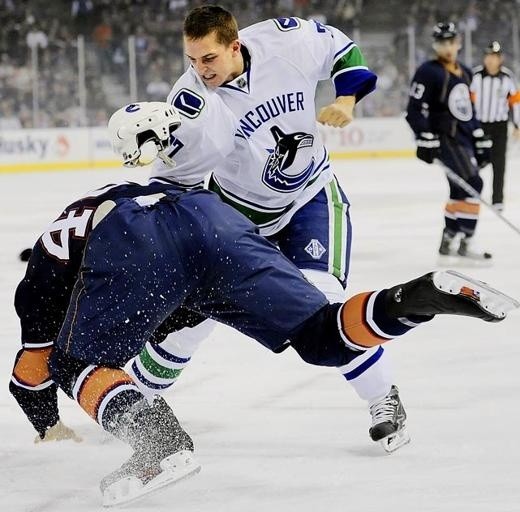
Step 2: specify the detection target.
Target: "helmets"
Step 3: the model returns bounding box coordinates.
[431,20,462,52]
[483,40,504,54]
[107,101,181,168]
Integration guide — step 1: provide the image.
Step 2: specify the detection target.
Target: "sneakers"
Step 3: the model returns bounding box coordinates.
[100,394,194,496]
[439,227,457,256]
[369,384,406,442]
[457,235,471,256]
[382,271,508,323]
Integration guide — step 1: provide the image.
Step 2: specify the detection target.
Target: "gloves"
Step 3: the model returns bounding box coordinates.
[473,134,493,170]
[36,423,83,443]
[416,132,441,163]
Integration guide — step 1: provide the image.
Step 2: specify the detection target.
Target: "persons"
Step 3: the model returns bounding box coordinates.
[1,0,519,127]
[9,176,520,508]
[468,41,520,212]
[106,4,411,453]
[405,22,492,260]
